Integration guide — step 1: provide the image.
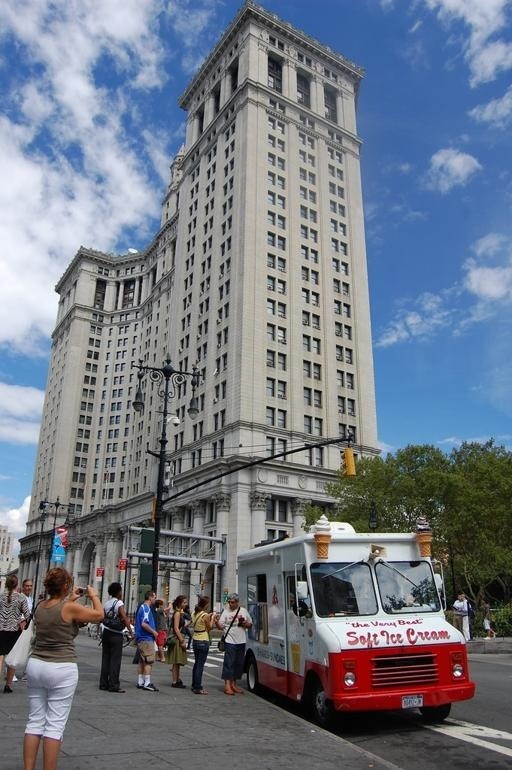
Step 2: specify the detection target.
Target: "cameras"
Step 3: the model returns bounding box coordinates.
[78,588,87,596]
[237,617,245,626]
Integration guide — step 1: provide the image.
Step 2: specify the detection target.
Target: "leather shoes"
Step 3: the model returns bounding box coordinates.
[4,684,12,693]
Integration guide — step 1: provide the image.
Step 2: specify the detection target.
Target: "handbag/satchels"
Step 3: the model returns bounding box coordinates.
[218,638,226,652]
[104,610,125,631]
[4,618,37,672]
[165,634,177,646]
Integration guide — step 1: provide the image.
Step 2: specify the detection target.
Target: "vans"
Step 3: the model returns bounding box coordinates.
[233,513,476,724]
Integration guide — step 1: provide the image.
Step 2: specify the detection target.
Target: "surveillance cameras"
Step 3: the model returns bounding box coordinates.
[172,418,180,426]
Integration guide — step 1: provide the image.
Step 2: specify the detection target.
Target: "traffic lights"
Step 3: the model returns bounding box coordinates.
[340,448,356,477]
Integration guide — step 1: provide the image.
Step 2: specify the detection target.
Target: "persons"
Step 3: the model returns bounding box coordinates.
[452,592,496,642]
[24,569,104,770]
[134,591,253,695]
[0,568,34,693]
[99,583,135,693]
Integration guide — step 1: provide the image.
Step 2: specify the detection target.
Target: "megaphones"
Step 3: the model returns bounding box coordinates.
[368,542,387,559]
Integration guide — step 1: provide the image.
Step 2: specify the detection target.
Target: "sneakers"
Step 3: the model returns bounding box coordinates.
[172,678,185,688]
[137,680,159,691]
[5,670,18,682]
[192,686,208,695]
[100,687,125,693]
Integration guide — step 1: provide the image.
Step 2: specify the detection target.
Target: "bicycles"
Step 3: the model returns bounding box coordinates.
[90,621,131,648]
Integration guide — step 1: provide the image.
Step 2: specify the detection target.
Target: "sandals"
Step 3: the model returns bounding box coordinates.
[224,685,243,695]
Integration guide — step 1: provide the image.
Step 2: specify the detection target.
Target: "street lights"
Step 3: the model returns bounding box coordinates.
[31,508,49,611]
[38,495,72,600]
[129,352,207,665]
